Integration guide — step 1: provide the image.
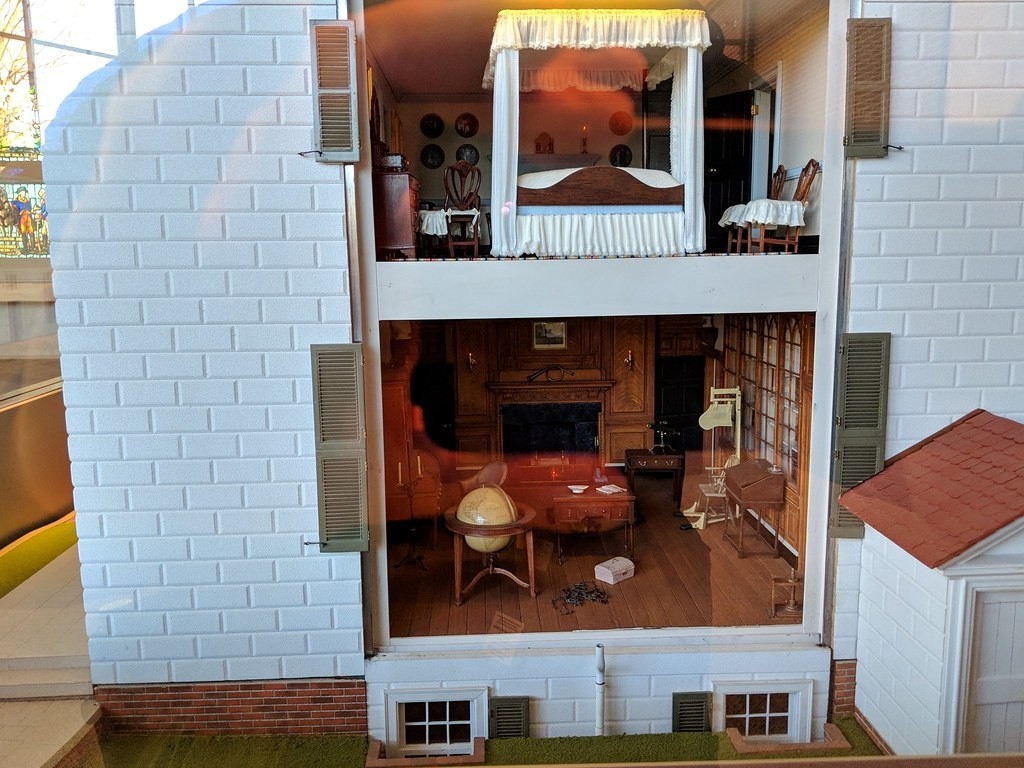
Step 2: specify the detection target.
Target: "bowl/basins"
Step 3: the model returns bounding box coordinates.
[566,484,589,494]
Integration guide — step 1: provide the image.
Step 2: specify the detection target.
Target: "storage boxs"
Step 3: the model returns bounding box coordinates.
[594,556,635,585]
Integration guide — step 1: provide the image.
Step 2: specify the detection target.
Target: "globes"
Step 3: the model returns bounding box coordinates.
[455,481,530,594]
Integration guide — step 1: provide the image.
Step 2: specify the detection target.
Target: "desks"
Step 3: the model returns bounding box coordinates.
[549,485,637,566]
[624,448,684,516]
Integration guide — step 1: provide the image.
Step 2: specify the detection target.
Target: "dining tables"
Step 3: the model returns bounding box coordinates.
[416,208,479,259]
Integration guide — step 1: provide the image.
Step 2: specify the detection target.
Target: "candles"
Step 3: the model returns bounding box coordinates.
[417,455,421,477]
[582,126,587,138]
[398,461,402,486]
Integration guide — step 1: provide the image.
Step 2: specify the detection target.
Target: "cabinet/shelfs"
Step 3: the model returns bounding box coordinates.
[717,311,816,580]
[452,315,655,475]
[371,171,423,259]
[380,339,457,522]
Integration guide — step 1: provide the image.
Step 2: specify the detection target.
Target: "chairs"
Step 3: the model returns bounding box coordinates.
[736,159,819,254]
[422,160,482,258]
[461,192,481,259]
[717,165,787,254]
[673,453,742,530]
[419,201,443,258]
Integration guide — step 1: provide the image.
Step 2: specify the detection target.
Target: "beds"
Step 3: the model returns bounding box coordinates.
[515,165,686,257]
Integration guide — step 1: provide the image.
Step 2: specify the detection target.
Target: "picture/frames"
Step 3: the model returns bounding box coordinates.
[420,113,445,139]
[608,144,633,168]
[455,113,479,138]
[609,111,633,135]
[531,321,568,351]
[455,144,480,166]
[420,144,445,169]
